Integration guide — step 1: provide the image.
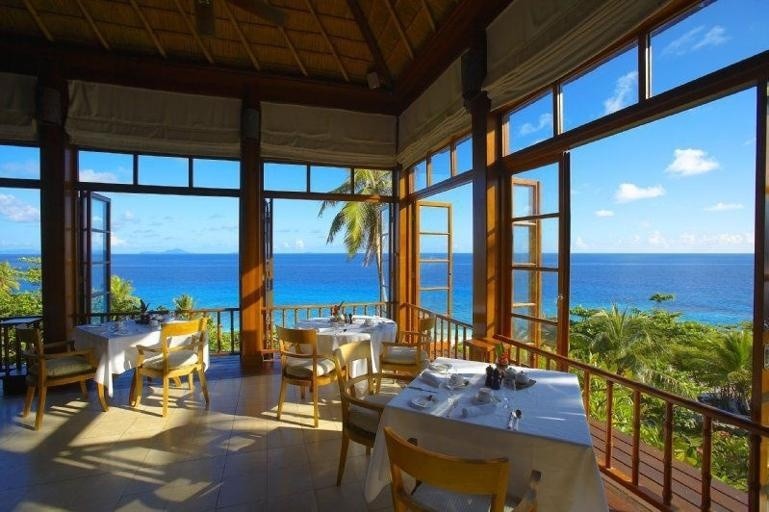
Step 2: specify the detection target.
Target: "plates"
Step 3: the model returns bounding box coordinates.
[411,397,439,407]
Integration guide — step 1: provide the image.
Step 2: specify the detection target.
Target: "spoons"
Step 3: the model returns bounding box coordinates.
[513,410,521,431]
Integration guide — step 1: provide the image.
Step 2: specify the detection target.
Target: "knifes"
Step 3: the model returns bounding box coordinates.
[408,387,438,393]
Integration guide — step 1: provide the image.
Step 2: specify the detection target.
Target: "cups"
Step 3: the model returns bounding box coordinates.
[479,388,496,404]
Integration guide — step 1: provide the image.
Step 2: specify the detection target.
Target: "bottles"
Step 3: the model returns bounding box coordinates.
[517,371,529,384]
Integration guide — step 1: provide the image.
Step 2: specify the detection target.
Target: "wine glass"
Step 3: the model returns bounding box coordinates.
[499,378,516,411]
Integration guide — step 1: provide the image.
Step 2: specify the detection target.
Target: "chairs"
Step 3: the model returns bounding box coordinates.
[18,316,213,431]
[273,314,437,429]
[329,340,542,512]
